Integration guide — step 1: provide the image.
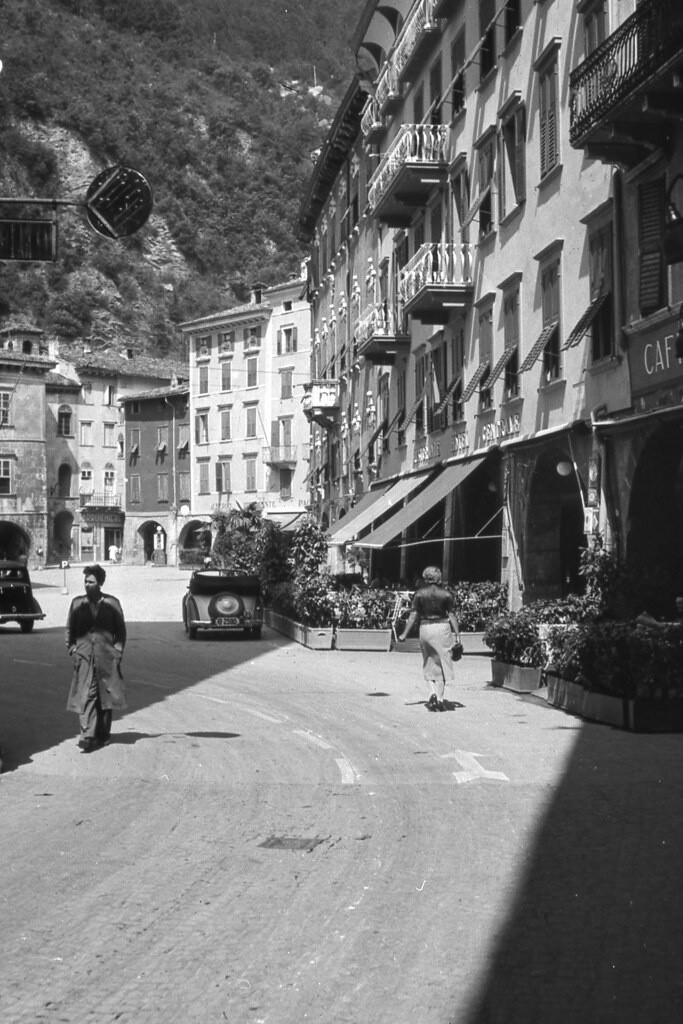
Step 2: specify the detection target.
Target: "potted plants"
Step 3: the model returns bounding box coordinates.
[254,515,683,734]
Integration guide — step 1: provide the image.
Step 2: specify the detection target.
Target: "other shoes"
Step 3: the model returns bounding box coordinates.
[78,737,94,753]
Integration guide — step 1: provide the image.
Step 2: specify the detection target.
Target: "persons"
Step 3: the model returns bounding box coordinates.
[108,544,119,562]
[398,565,461,711]
[66,564,126,750]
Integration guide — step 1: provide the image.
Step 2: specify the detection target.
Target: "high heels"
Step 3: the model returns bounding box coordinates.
[436,701,447,711]
[425,694,438,712]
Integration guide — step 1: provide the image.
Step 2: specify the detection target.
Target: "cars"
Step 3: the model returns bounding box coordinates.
[0,561,46,631]
[182,567,263,639]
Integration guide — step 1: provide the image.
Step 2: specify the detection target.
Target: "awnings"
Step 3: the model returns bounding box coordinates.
[127,443,138,454]
[325,481,394,537]
[176,441,188,449]
[344,447,359,464]
[432,346,516,417]
[154,442,165,451]
[303,462,329,484]
[560,296,609,353]
[353,452,491,551]
[516,323,562,375]
[357,398,423,460]
[327,468,436,544]
